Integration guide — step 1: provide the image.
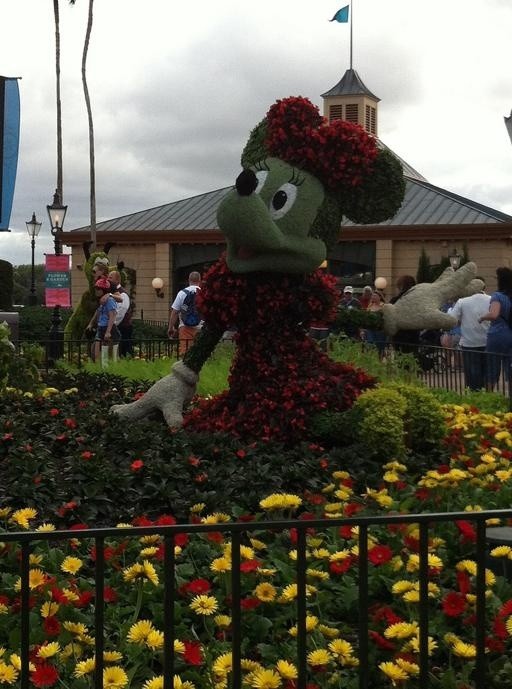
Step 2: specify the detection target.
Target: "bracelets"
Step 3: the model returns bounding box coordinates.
[108,292,113,298]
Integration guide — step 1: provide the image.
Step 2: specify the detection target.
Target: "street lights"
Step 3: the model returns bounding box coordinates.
[448,246,461,274]
[47,189,67,332]
[25,209,43,292]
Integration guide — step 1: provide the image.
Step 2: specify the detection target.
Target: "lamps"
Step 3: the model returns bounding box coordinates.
[374,277,387,298]
[151,277,165,299]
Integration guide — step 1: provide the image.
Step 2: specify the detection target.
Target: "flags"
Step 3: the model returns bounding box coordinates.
[327,5,349,24]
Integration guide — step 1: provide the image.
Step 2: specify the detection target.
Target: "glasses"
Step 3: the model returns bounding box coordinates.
[91,269,100,273]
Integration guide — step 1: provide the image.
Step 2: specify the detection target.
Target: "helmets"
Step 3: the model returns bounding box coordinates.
[95,279,111,289]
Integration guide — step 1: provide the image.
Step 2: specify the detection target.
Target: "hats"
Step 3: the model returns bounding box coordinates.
[343,286,353,294]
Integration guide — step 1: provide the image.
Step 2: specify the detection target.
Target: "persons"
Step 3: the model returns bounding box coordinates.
[90,261,109,281]
[165,270,204,360]
[90,269,134,358]
[86,277,122,363]
[340,265,511,399]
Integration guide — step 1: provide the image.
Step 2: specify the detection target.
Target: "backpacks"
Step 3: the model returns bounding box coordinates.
[180,289,201,326]
